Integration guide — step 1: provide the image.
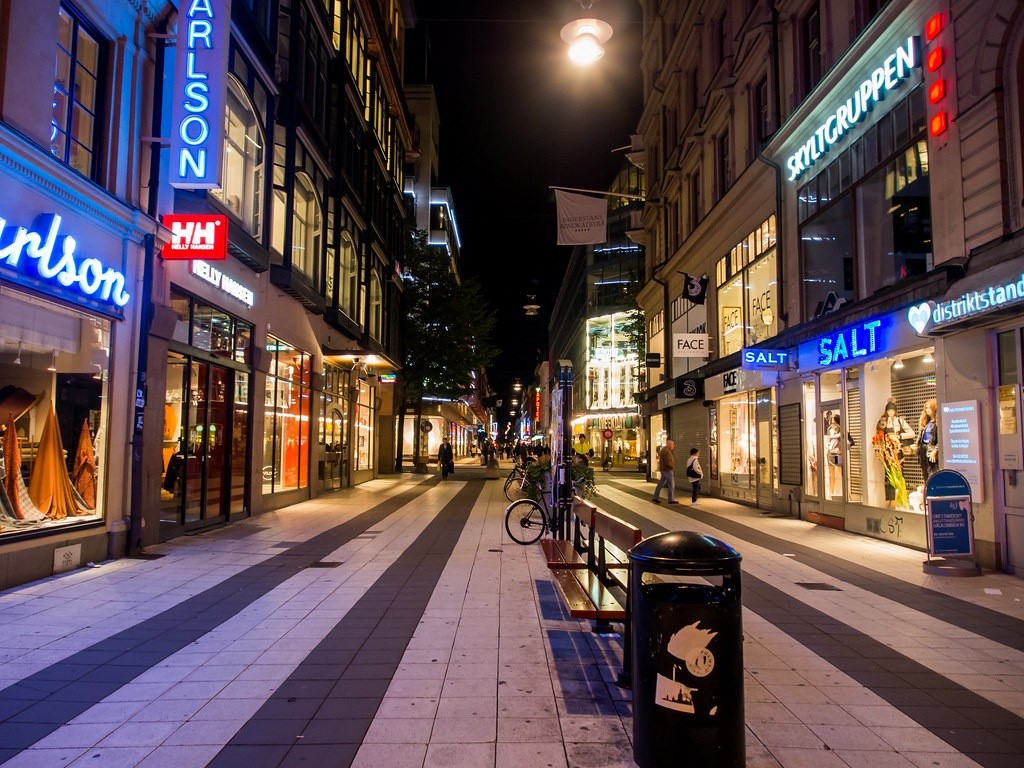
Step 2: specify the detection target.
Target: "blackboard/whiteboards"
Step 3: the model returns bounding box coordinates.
[926,495,974,557]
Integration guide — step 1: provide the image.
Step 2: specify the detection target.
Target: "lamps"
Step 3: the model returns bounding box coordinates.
[894,356,904,368]
[923,351,934,362]
[871,364,880,371]
[560,0,613,65]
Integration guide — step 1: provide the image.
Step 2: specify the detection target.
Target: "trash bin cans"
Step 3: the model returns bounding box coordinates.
[627,531,746,768]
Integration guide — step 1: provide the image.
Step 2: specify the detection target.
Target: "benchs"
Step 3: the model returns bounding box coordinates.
[538,495,644,687]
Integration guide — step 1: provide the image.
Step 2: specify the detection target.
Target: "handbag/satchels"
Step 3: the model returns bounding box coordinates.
[449,461,455,474]
[437,461,441,472]
[898,418,914,446]
[687,459,701,478]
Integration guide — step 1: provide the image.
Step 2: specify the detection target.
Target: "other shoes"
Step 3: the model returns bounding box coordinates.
[651,499,661,503]
[692,501,702,506]
[668,500,679,504]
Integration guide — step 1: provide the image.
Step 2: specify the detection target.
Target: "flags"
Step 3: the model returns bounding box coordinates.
[556,189,607,245]
[682,275,709,306]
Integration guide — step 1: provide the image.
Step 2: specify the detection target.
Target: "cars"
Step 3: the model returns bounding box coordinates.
[641,452,647,471]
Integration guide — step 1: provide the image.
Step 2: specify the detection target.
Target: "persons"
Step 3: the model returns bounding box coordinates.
[437,438,453,480]
[651,440,679,505]
[824,410,855,496]
[469,441,551,467]
[687,448,703,506]
[914,399,938,509]
[571,434,594,468]
[876,401,915,508]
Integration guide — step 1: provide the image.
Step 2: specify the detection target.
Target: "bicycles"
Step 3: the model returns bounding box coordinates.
[504,461,535,502]
[602,453,613,471]
[505,477,595,546]
[502,458,534,492]
